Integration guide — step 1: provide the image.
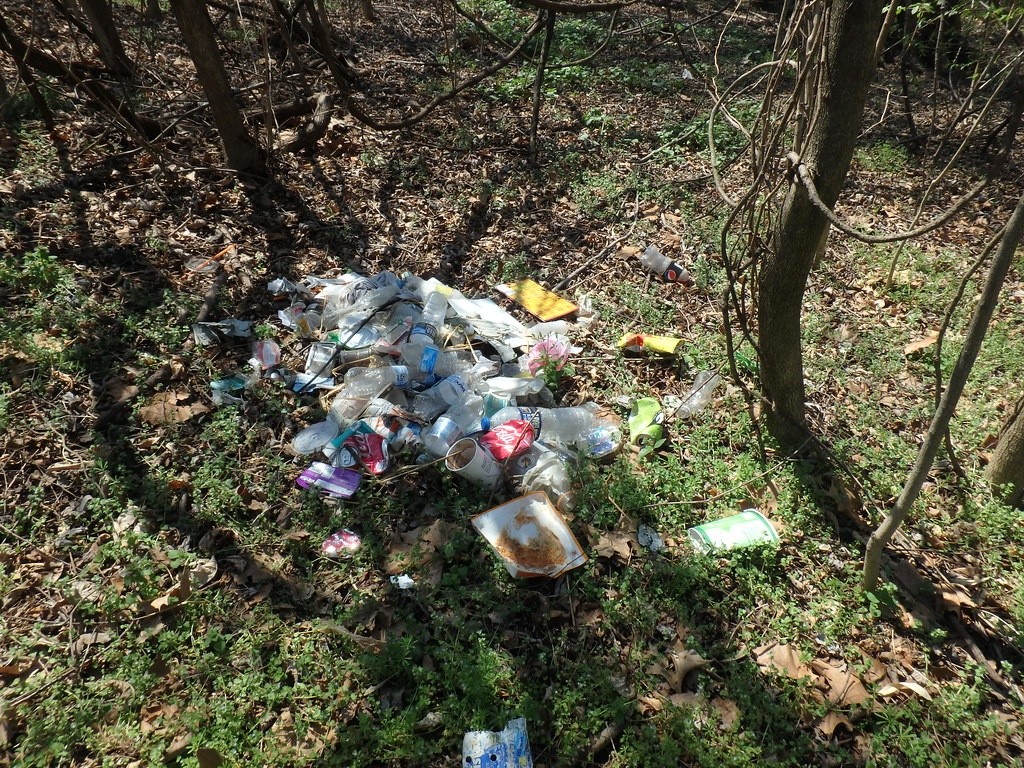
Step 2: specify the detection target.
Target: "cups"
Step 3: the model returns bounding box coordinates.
[688,508,780,555]
[445,437,505,493]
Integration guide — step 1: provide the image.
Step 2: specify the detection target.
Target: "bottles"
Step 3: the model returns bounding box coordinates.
[675,370,721,419]
[640,246,693,283]
[301,289,599,513]
